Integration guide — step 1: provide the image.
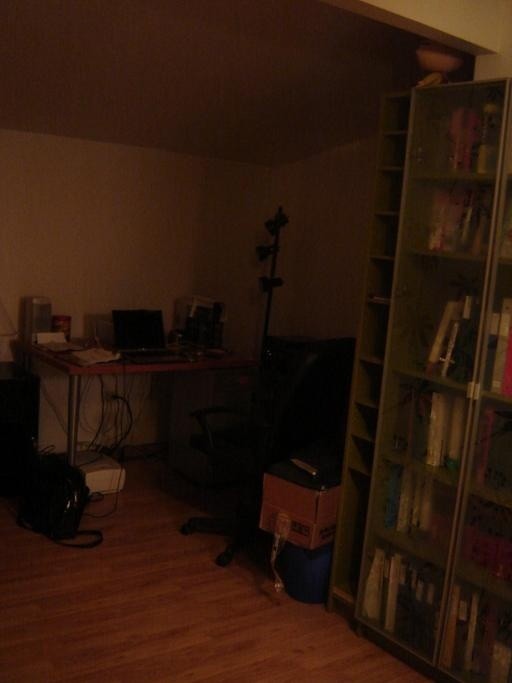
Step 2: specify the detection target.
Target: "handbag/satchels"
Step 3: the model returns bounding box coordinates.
[18,461,87,539]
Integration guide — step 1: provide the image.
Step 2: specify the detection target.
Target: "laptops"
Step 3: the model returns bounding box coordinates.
[111,308,187,364]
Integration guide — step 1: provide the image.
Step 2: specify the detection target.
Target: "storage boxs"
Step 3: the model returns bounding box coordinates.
[258,473,342,552]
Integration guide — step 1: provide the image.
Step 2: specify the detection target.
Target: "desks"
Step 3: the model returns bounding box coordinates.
[10,340,259,474]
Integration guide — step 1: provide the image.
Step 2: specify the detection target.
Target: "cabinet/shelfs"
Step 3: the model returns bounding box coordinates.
[326,76,512,683]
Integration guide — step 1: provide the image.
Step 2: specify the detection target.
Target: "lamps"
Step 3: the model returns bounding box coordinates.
[254,206,290,337]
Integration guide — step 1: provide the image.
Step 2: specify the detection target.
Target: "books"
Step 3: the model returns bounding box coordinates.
[448,101,503,173]
[442,581,512,681]
[420,295,512,399]
[59,346,122,366]
[392,381,512,487]
[361,541,447,663]
[384,465,512,584]
[427,182,512,258]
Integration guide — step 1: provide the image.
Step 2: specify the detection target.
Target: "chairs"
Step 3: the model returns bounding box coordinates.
[179,332,356,568]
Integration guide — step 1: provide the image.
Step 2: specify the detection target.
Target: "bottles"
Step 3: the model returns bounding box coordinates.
[475,102,500,177]
